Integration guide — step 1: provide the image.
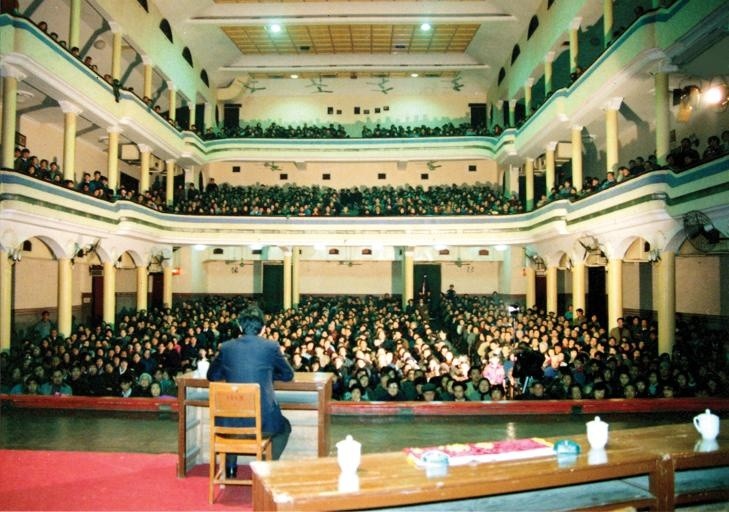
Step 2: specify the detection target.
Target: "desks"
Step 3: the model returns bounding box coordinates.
[249,420,729,512]
[173,373,334,478]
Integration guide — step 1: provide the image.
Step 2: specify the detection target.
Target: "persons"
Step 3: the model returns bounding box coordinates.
[11,130,729,218]
[515,4,646,128]
[206,306,294,478]
[186,123,502,143]
[1,294,729,401]
[38,20,177,128]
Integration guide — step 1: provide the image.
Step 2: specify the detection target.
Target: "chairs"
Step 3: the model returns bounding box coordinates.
[208,381,272,504]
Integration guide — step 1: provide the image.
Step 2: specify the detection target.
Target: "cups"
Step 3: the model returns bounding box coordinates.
[695,438,719,453]
[587,449,606,465]
[337,472,361,497]
[586,415,610,449]
[197,362,210,376]
[424,450,450,479]
[693,409,721,438]
[336,433,363,470]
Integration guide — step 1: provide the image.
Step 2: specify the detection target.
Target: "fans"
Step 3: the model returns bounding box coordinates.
[443,74,464,91]
[311,76,333,94]
[305,79,327,88]
[146,251,171,272]
[228,247,256,266]
[427,161,440,170]
[684,210,729,253]
[339,250,362,267]
[574,236,606,260]
[239,75,266,92]
[71,237,102,266]
[445,247,471,267]
[266,162,282,171]
[366,74,394,94]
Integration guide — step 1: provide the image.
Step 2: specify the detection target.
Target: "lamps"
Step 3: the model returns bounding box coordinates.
[673,73,729,119]
